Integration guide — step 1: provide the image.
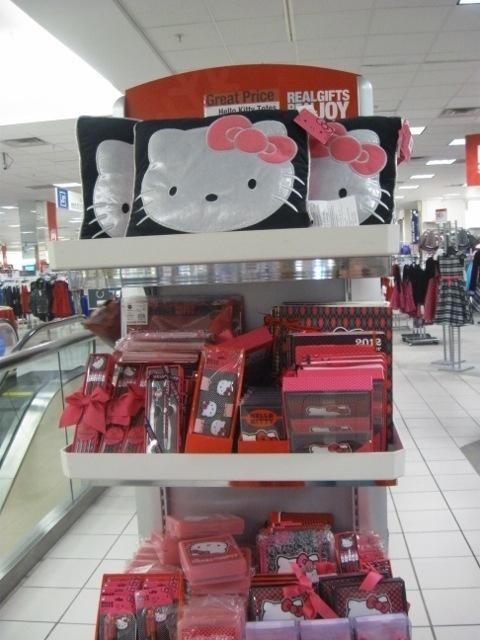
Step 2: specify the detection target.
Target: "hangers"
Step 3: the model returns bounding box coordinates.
[393,227,480,268]
[0,274,72,286]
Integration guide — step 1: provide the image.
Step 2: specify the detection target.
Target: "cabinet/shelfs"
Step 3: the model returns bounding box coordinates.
[47,224,405,639]
